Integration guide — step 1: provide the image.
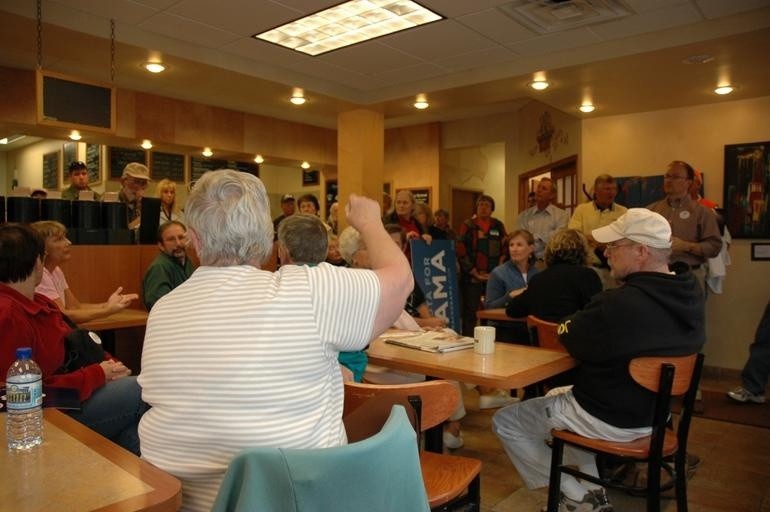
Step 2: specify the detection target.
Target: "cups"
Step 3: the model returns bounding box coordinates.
[474,326,496,354]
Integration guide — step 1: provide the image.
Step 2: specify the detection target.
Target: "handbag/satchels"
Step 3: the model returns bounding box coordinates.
[63,326,104,372]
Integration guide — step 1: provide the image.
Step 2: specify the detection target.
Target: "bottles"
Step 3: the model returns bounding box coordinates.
[5,346,44,451]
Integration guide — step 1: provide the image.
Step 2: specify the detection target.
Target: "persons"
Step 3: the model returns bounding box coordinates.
[30,188,48,201]
[23,218,139,324]
[1,222,154,456]
[721,301,769,406]
[116,161,152,244]
[135,169,418,512]
[141,153,726,452]
[487,208,709,510]
[59,161,103,202]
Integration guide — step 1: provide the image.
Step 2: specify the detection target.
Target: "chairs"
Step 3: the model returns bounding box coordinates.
[544,352,703,512]
[526,314,566,398]
[211,405,430,512]
[341,381,481,511]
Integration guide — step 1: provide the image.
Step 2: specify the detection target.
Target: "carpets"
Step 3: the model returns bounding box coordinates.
[670,389,769,429]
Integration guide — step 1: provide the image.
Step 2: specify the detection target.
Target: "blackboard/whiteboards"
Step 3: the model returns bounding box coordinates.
[394,186,433,212]
[149,151,189,185]
[85,142,102,187]
[190,155,259,187]
[108,145,149,180]
[302,170,320,186]
[42,149,61,192]
[34,66,118,136]
[63,142,79,186]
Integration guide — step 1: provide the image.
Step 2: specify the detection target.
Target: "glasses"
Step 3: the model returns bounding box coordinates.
[607,242,634,250]
[71,160,82,166]
[662,174,690,181]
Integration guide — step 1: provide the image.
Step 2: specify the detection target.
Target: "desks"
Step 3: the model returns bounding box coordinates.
[364,327,576,512]
[0,389,183,512]
[476,309,537,328]
[63,303,150,359]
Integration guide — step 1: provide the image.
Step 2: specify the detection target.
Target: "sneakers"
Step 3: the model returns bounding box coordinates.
[688,454,700,466]
[727,386,765,403]
[542,490,601,512]
[442,431,464,449]
[693,399,703,414]
[480,391,520,409]
[593,488,614,512]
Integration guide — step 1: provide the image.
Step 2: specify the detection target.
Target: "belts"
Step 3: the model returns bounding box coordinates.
[689,265,700,270]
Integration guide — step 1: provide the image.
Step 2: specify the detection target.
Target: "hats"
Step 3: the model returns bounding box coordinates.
[281,194,295,201]
[592,207,672,249]
[121,162,151,181]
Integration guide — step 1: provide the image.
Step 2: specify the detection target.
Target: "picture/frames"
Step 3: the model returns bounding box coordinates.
[722,141,770,239]
